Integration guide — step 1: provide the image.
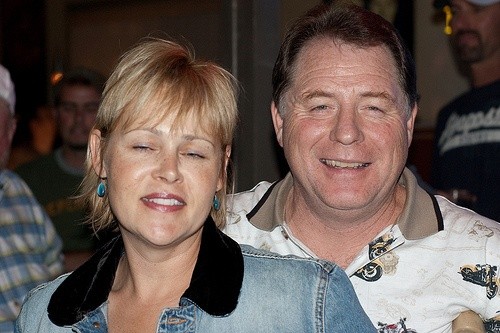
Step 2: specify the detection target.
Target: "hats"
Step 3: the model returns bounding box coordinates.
[0,64,16,115]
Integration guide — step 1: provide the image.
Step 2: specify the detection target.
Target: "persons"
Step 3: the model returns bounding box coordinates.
[221,0,500,333]
[16,37,379,333]
[433,0,500,223]
[0,65,109,333]
